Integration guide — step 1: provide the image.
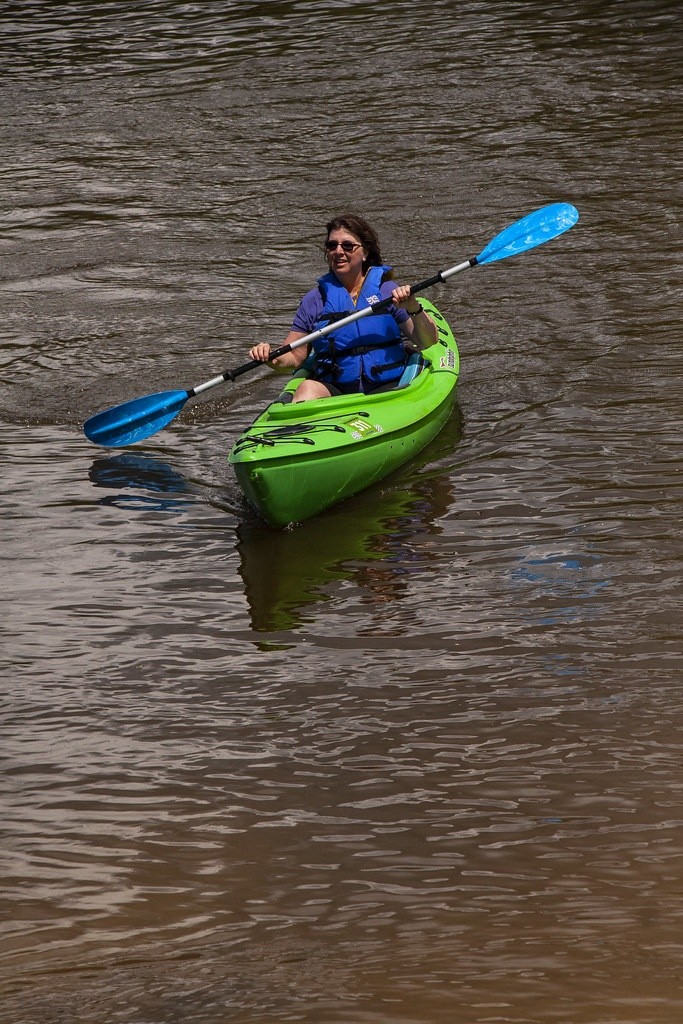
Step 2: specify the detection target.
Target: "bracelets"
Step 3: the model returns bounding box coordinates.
[405,304,424,317]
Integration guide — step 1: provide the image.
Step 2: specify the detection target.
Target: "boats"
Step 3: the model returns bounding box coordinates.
[226,293,461,530]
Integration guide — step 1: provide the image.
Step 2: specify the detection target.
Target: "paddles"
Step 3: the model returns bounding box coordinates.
[84,199,581,447]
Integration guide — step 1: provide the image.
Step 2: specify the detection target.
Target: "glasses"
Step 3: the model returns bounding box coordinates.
[324,241,362,252]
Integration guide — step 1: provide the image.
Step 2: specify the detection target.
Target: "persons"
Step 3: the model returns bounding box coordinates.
[250,213,439,402]
